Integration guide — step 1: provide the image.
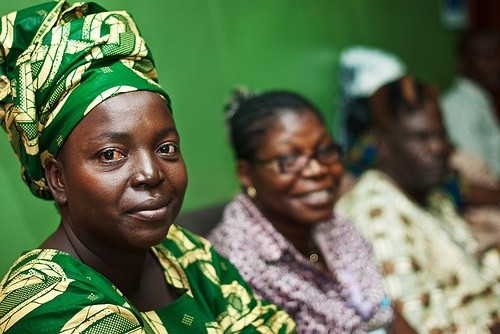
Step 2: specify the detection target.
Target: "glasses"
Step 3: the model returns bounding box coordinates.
[250,144,344,173]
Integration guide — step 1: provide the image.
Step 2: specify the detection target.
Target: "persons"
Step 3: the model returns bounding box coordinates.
[334,24,500,334]
[0,0,299,334]
[206,86,420,334]
